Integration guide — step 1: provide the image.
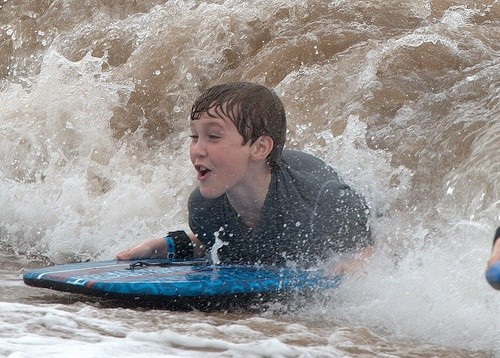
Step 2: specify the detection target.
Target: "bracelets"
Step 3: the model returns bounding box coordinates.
[164,237,177,260]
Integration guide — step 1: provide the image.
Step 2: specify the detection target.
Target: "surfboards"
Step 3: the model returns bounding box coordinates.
[22,259,338,309]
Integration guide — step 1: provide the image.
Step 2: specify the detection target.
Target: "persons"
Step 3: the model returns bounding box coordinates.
[116,83,376,281]
